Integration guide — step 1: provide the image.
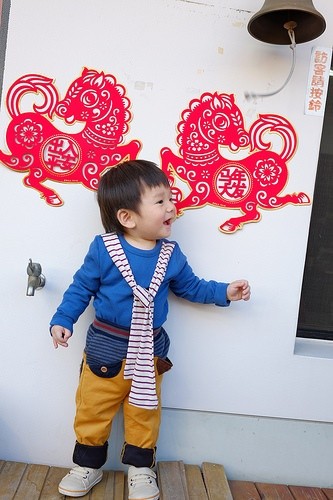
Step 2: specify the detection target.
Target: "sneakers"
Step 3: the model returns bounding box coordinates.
[128,466,160,500]
[58,464,103,497]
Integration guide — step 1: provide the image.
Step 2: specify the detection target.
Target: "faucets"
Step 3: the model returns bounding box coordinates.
[26,259,46,296]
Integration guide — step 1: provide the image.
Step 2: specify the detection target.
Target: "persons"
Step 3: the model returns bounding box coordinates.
[46,159,251,500]
